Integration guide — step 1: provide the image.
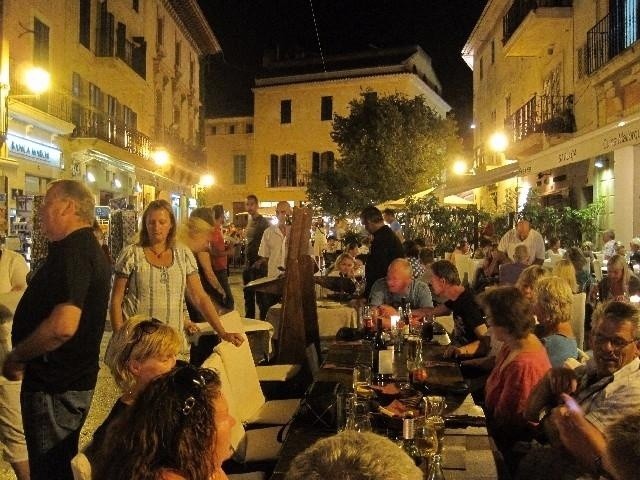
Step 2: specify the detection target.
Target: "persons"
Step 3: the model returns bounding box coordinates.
[0,179,639,480]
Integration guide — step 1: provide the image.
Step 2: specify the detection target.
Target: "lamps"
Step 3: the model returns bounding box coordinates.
[594,156,604,169]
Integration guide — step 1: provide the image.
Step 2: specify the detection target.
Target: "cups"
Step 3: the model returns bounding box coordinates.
[337,393,358,417]
[353,366,371,388]
[406,334,422,372]
[423,322,433,340]
[427,396,445,416]
[353,416,373,432]
[415,421,438,454]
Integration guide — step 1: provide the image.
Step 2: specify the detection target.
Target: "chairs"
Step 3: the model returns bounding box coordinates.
[70,443,114,480]
[568,292,586,350]
[212,335,301,425]
[213,308,301,387]
[199,352,293,463]
[591,259,605,283]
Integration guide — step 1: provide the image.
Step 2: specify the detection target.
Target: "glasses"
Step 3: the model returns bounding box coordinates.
[172,360,205,435]
[120,317,164,365]
[482,315,496,329]
[592,332,636,347]
[160,265,167,281]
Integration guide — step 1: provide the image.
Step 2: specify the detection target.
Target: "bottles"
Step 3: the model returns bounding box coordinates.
[371,318,385,371]
[402,409,421,466]
[398,295,410,334]
[363,306,372,330]
[428,455,446,480]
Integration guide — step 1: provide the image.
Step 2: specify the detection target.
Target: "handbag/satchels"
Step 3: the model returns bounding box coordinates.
[296,382,345,433]
